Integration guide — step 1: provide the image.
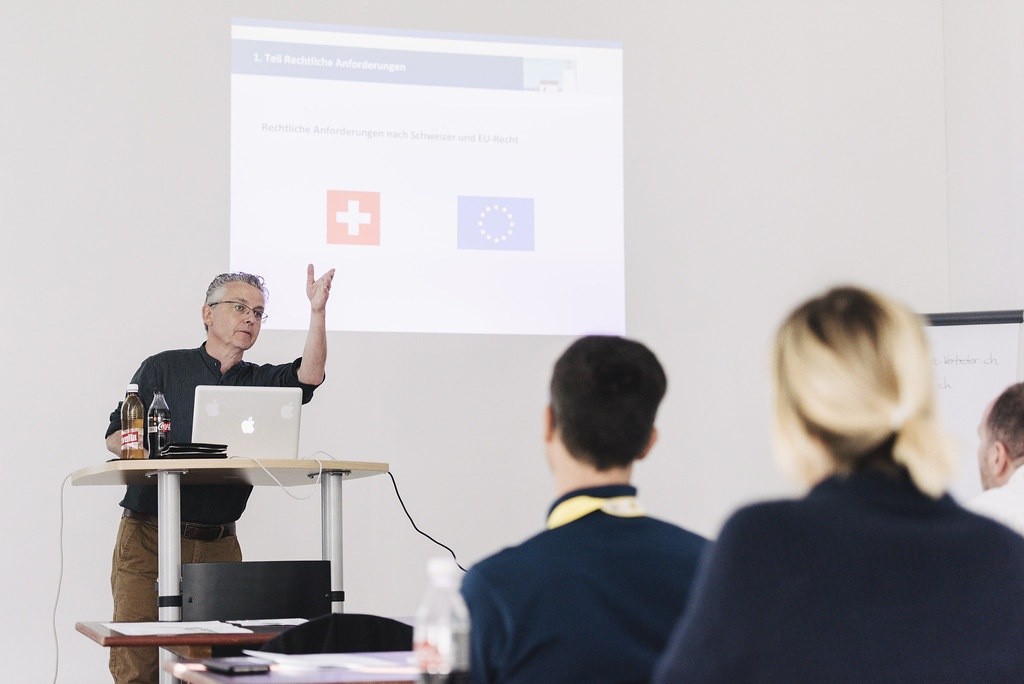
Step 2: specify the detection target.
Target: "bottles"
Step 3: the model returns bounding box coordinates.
[121,383,145,459]
[147,386,172,459]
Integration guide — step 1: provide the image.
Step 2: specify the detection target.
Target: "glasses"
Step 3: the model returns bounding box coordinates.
[210,301,268,324]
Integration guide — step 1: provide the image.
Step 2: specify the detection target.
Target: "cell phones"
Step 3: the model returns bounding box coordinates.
[201,656,270,675]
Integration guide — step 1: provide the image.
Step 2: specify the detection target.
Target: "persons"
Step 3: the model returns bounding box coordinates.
[103,261,335,684]
[448,335,715,684]
[653,285,1024,684]
[964,380,1024,538]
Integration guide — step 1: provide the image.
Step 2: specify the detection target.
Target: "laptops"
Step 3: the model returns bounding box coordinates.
[191,385,303,460]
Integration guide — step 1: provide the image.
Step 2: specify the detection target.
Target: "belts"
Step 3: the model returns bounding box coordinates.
[123,509,236,540]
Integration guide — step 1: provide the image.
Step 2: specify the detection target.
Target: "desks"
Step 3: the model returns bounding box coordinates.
[161,650,415,684]
[75,618,309,659]
[70,459,390,684]
